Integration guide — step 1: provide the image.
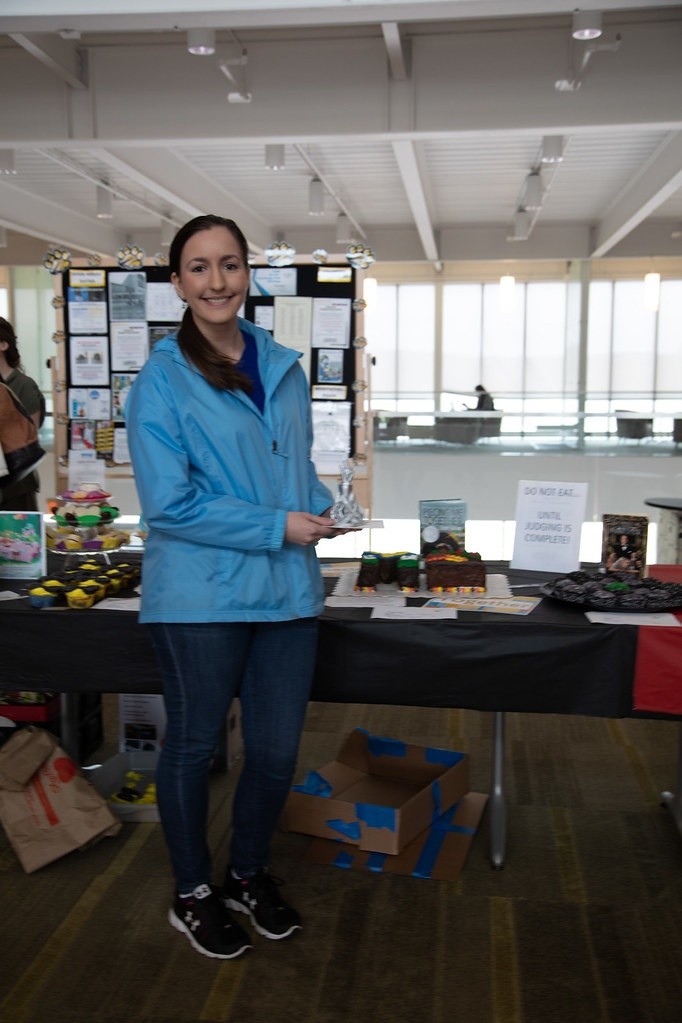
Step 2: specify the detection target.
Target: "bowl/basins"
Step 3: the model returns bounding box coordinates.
[29,558,141,610]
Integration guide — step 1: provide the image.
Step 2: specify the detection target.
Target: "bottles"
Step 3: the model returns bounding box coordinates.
[331,459,364,528]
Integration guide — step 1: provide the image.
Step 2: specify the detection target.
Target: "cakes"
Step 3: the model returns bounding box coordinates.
[424,548,486,595]
[355,551,422,593]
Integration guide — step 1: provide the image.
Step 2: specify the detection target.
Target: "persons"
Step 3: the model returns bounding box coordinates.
[615,535,631,560]
[125,216,360,961]
[467,386,494,411]
[0,318,46,513]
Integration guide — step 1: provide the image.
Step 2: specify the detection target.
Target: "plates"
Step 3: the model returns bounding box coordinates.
[539,580,681,613]
[47,490,123,555]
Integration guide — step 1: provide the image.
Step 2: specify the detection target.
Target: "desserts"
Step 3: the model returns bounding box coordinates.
[28,562,138,607]
[553,572,682,607]
[30,484,147,550]
[107,771,156,803]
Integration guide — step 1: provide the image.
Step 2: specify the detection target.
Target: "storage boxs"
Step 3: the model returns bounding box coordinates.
[0,688,103,767]
[118,690,246,773]
[279,728,473,856]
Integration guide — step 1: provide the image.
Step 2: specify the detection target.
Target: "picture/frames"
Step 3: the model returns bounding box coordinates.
[603,514,649,578]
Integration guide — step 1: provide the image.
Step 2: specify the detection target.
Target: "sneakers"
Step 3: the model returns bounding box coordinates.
[217,867,305,939]
[172,884,254,960]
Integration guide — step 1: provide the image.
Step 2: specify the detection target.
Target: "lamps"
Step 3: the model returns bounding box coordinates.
[514,211,531,241]
[160,220,176,247]
[336,216,353,244]
[307,180,325,216]
[96,187,113,220]
[265,145,284,169]
[186,28,216,56]
[0,149,15,175]
[572,9,603,40]
[542,134,563,163]
[525,175,543,211]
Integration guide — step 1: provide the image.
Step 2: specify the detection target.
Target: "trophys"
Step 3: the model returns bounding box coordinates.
[328,457,363,527]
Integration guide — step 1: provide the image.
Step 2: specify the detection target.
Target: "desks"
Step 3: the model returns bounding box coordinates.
[644,496,682,565]
[0,557,682,870]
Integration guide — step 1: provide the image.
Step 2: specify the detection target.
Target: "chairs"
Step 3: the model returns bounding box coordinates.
[616,410,655,447]
